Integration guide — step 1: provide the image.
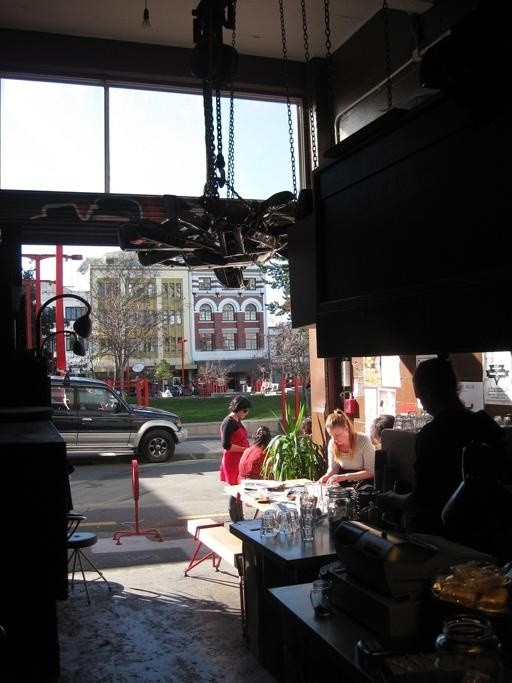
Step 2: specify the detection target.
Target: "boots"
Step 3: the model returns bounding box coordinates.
[227,507,245,523]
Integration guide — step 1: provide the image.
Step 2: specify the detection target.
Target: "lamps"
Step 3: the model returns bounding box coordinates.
[119,0,301,288]
[36,295,94,358]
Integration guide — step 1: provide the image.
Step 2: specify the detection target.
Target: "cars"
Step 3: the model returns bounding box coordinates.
[48,375,188,462]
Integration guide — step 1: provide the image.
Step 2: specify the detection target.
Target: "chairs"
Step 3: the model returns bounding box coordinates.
[67,514,111,605]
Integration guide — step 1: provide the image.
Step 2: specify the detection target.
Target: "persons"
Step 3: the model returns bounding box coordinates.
[237,424,278,481]
[313,407,378,490]
[367,415,397,453]
[368,356,504,536]
[218,394,252,523]
[299,416,314,448]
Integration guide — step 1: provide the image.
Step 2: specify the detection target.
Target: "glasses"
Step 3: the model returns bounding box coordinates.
[240,408,249,413]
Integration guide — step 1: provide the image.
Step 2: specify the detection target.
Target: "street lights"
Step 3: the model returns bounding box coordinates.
[20,253,82,402]
[178,337,188,384]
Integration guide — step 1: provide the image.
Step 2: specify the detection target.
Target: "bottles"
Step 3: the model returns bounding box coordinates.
[310,580,335,617]
[326,489,353,532]
[435,615,503,683]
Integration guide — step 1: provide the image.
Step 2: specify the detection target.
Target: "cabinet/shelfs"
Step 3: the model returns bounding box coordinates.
[0,333,68,683]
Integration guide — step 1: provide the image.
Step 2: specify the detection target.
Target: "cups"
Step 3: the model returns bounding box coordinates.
[260,495,318,541]
[391,414,433,434]
[493,415,511,428]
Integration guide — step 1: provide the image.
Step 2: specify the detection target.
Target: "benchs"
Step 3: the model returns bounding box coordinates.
[184,518,245,576]
